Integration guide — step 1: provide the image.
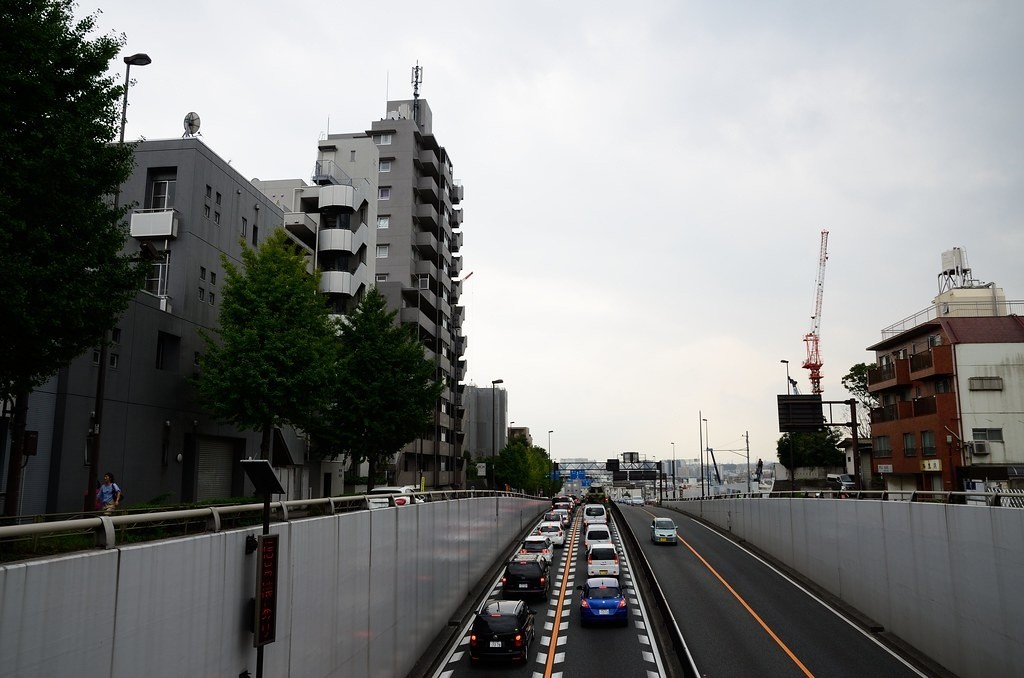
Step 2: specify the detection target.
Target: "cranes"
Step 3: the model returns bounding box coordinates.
[802,228,830,394]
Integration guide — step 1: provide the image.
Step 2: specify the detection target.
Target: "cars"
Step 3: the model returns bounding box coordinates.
[518,536,556,567]
[650,517,679,546]
[585,543,620,577]
[576,577,629,626]
[584,524,614,547]
[612,496,656,507]
[538,521,567,547]
[543,494,579,526]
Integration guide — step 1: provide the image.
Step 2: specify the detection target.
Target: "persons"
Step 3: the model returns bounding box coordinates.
[757,459,763,474]
[840,486,850,499]
[96,472,121,515]
[83,478,102,511]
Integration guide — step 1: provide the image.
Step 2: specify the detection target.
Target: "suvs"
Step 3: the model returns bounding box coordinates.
[500,555,553,601]
[366,487,425,508]
[469,598,537,665]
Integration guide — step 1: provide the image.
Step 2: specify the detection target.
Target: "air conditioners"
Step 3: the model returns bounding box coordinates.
[972,440,990,454]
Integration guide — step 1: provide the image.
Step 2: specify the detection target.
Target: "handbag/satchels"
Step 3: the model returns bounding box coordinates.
[112,484,125,503]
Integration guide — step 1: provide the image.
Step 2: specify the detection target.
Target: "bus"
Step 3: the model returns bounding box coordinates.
[584,484,609,507]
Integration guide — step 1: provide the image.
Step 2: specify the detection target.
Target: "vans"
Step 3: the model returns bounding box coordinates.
[827,473,855,488]
[581,504,609,528]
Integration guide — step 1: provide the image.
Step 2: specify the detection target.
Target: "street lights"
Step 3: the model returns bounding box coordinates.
[780,359,789,395]
[81,53,153,537]
[548,430,554,476]
[491,379,504,460]
[671,442,675,498]
[703,417,710,496]
[241,458,287,678]
